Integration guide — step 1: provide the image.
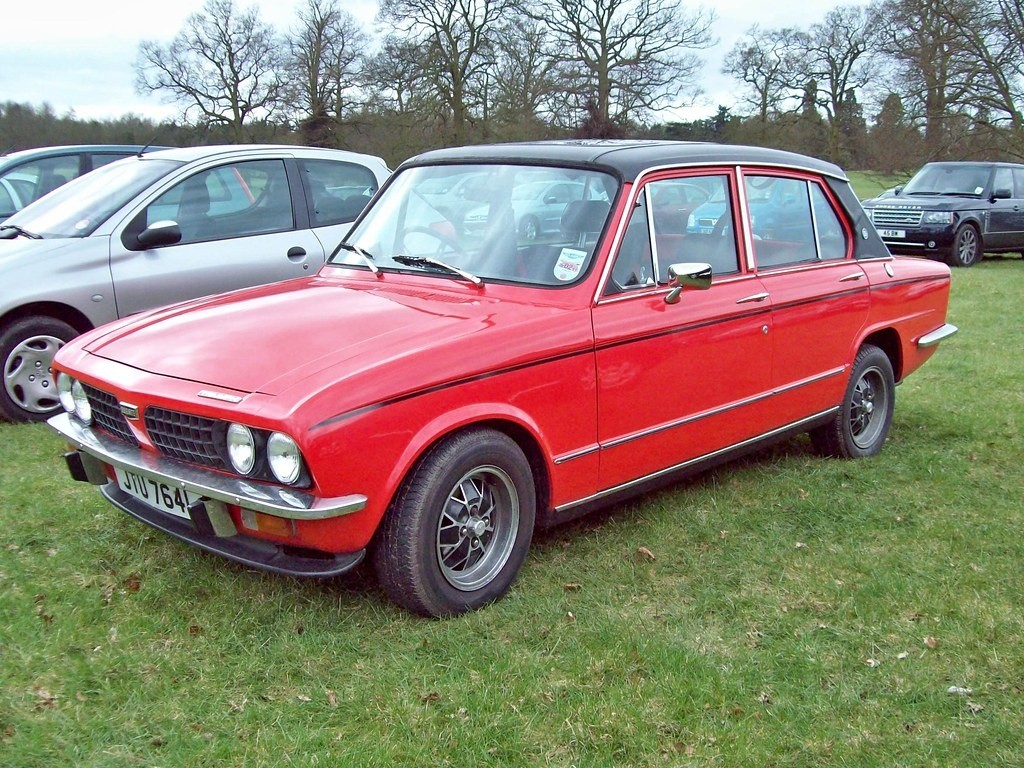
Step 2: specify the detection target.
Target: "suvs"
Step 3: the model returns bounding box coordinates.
[853,161,1024,266]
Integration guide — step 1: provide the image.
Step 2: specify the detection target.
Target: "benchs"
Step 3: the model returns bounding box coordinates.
[646,233,805,271]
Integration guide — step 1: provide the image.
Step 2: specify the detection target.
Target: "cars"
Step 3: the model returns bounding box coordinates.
[368,168,839,244]
[0,143,256,228]
[0,144,462,422]
[43,135,958,617]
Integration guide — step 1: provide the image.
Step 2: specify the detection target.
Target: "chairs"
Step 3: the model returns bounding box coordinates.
[526,198,611,284]
[175,182,219,240]
[43,175,66,195]
